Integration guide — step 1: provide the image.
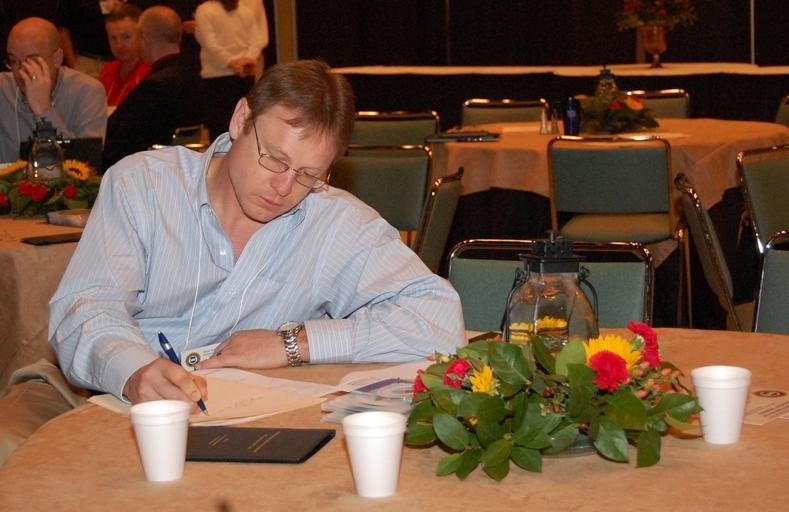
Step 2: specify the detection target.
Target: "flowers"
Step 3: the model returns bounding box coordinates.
[0,159,106,222]
[403,314,702,482]
[572,87,661,133]
[617,0,697,30]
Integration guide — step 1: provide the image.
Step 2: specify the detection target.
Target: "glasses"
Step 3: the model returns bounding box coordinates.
[250,107,333,191]
[2,47,61,72]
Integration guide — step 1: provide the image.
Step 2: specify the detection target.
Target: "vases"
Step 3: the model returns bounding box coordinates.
[639,26,667,69]
[532,433,600,457]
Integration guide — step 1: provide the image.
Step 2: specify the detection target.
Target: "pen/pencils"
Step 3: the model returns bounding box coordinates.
[157,330,209,418]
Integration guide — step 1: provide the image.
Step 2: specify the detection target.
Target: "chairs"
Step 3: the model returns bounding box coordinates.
[747,229,789,333]
[569,89,689,130]
[543,137,693,330]
[459,97,550,128]
[735,143,788,311]
[329,145,432,256]
[437,237,655,332]
[342,108,437,150]
[413,166,469,275]
[672,171,789,331]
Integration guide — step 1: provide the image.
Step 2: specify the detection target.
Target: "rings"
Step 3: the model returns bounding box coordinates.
[31,76,37,80]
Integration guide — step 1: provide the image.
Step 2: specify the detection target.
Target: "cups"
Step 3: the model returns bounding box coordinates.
[339,409,408,500]
[560,96,582,136]
[689,363,753,447]
[127,399,191,485]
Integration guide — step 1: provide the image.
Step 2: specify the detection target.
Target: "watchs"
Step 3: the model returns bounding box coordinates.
[276,320,304,367]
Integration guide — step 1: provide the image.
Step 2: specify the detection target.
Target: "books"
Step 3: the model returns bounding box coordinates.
[424,132,499,143]
[20,232,82,245]
[186,426,336,463]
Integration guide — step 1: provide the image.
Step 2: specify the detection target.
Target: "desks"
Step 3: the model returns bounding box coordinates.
[1,210,110,394]
[428,117,787,331]
[0,324,437,512]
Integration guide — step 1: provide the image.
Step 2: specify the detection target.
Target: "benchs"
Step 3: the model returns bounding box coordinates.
[332,60,789,117]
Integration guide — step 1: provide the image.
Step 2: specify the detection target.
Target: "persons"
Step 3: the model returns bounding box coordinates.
[195,0,269,144]
[98,7,149,116]
[101,6,201,176]
[0,60,469,466]
[1,17,108,166]
[60,0,127,78]
